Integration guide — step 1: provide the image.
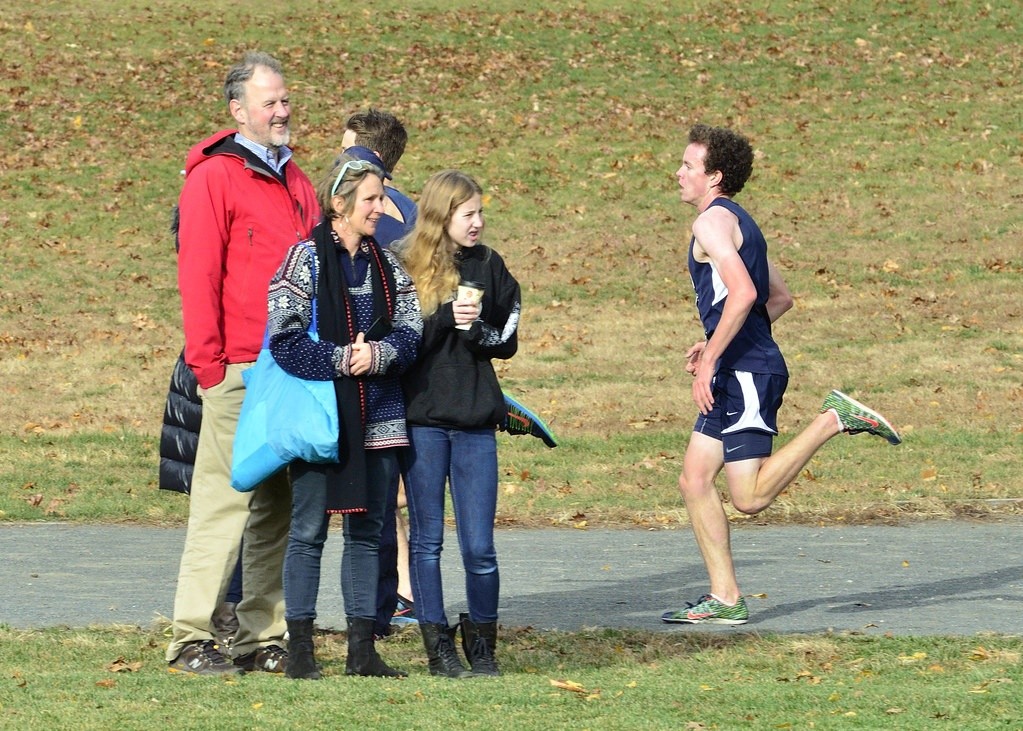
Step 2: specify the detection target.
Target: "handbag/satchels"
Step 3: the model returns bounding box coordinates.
[228,242,341,493]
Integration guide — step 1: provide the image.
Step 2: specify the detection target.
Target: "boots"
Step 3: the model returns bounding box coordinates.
[286,618,323,680]
[213,602,241,660]
[459,612,504,677]
[343,618,409,679]
[419,623,476,679]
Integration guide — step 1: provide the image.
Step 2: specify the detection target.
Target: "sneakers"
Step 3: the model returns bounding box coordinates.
[819,389,902,445]
[234,646,288,675]
[498,390,558,448]
[389,599,419,626]
[168,641,246,679]
[662,595,749,626]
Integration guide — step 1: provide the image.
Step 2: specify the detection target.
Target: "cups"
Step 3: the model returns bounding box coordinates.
[457,280,486,307]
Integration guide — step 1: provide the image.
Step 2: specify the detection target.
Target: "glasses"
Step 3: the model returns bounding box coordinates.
[331,160,372,196]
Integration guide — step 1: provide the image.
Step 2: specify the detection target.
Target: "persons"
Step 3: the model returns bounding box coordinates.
[388,168,521,680]
[658,120,902,628]
[265,144,425,685]
[156,205,246,660]
[340,107,560,626]
[162,48,323,682]
